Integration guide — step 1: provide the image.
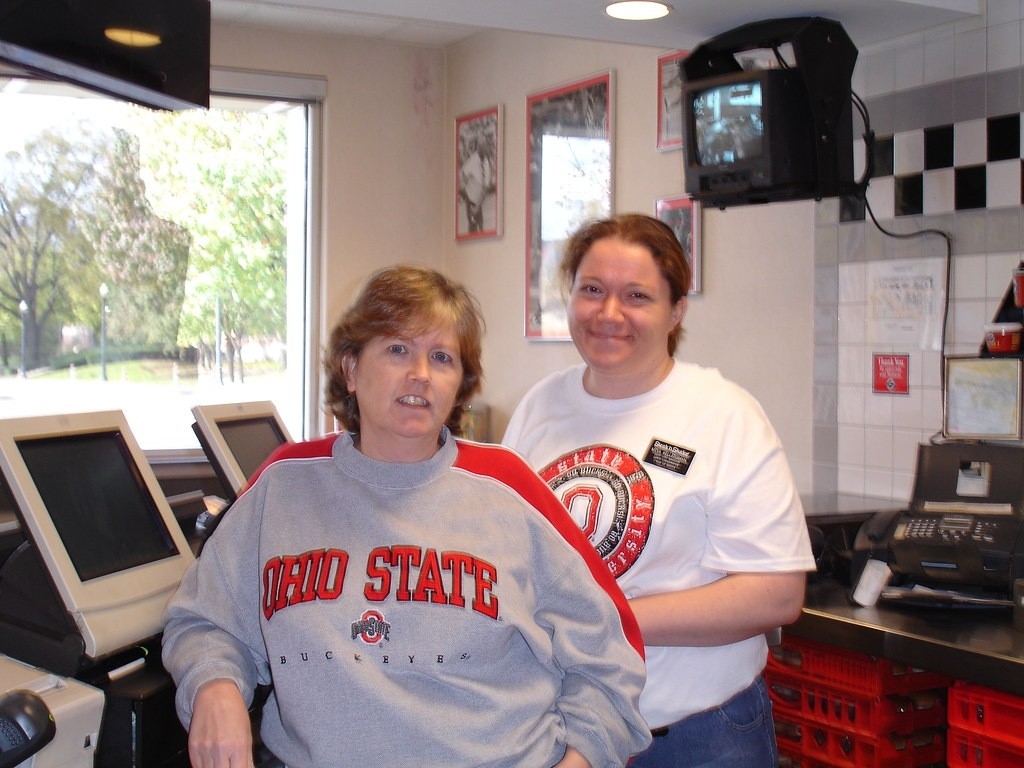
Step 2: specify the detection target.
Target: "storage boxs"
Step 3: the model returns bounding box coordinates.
[947,728,1024,768]
[760,669,949,739]
[948,679,1024,757]
[771,710,947,768]
[766,634,953,698]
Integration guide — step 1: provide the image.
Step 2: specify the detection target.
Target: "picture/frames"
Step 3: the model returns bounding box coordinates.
[454,102,504,243]
[522,68,617,342]
[652,193,702,294]
[656,48,691,152]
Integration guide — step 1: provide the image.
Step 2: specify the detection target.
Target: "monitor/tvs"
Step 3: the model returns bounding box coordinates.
[190,401,295,503]
[0,408,196,662]
[0,0,210,112]
[675,16,861,210]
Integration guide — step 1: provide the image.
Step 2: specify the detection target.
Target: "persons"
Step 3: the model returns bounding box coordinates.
[695,110,762,164]
[459,118,497,233]
[163,270,652,767]
[499,213,816,768]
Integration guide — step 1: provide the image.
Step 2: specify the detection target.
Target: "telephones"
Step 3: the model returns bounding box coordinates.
[854,441,1024,594]
